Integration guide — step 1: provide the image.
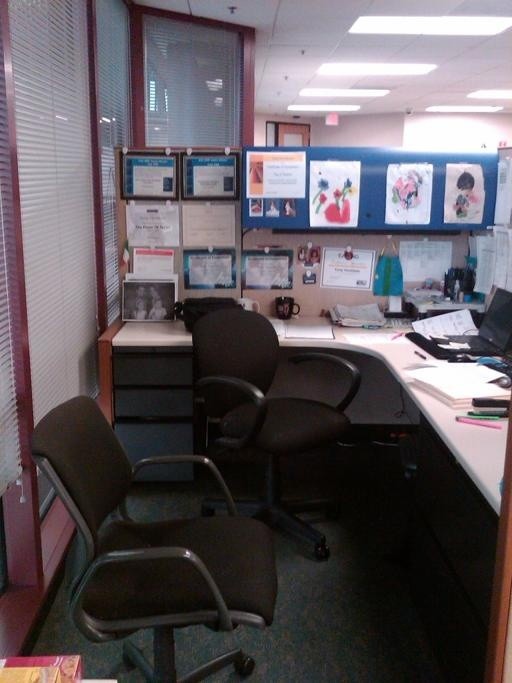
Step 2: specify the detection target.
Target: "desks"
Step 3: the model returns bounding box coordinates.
[97,314,509,517]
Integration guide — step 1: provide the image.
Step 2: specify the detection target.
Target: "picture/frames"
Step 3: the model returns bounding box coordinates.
[119,151,179,201]
[180,152,241,201]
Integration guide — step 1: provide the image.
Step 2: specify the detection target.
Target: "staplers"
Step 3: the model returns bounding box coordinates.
[467,397,510,417]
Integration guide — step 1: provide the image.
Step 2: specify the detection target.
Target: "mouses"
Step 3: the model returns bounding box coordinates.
[448,353,470,362]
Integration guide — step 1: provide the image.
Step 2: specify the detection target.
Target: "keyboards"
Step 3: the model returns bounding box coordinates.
[406,332,451,359]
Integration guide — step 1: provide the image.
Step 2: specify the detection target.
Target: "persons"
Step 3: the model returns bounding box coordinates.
[128,284,170,320]
[251,199,297,217]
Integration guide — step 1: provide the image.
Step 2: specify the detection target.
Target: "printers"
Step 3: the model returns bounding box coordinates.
[405,288,487,328]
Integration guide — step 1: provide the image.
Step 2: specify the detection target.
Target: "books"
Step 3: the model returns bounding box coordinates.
[411,373,510,402]
[414,383,471,409]
[330,303,385,328]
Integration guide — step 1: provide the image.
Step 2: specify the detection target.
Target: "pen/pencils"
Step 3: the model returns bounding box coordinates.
[392,332,404,340]
[415,351,426,359]
[455,416,504,431]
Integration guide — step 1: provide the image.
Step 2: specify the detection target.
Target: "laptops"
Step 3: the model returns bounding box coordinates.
[430,288,512,355]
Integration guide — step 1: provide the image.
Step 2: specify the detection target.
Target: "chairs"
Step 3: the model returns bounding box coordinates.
[192,308,360,562]
[29,395,278,683]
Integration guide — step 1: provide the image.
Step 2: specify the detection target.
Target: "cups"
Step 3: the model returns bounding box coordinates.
[273,295,302,320]
[236,297,261,314]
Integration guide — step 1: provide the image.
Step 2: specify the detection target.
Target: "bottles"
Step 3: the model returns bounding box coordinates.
[454,279,460,300]
